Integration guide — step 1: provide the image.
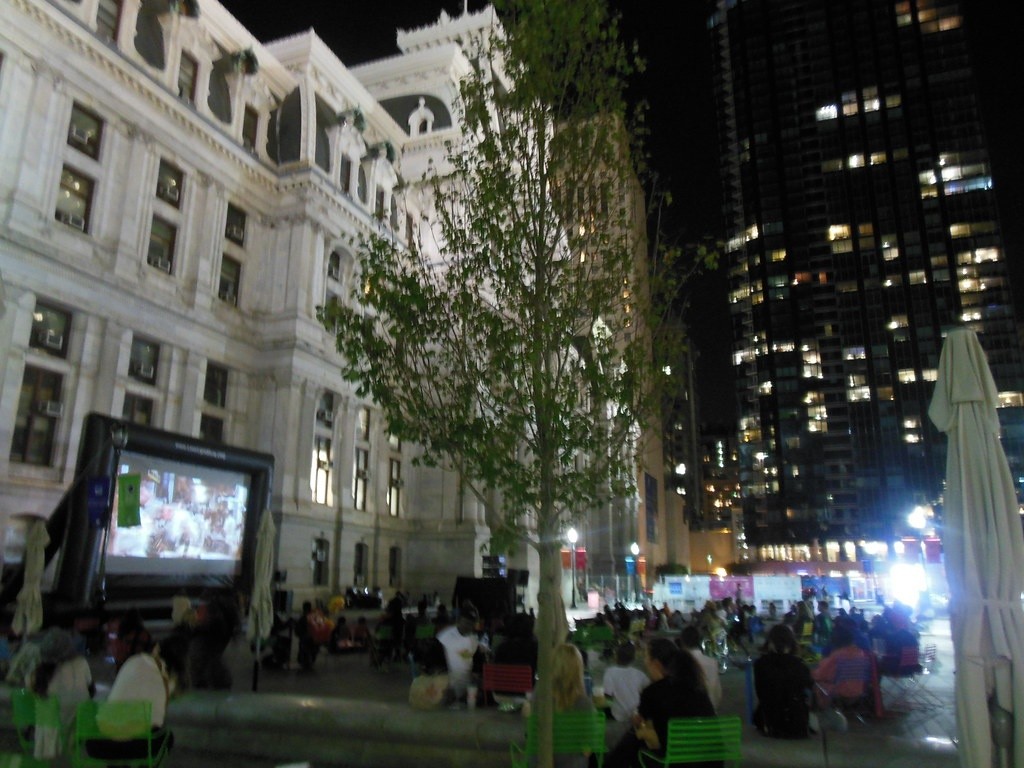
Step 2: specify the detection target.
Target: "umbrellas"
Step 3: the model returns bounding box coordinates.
[244,507,277,688]
[927,327,1024,768]
[10,520,46,648]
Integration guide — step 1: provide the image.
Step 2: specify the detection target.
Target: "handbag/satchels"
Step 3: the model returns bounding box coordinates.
[336,638,352,649]
[415,608,435,638]
[376,625,392,641]
[635,720,660,750]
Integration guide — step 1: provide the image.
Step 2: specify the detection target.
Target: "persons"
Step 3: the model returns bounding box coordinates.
[0,588,243,768]
[294,577,923,768]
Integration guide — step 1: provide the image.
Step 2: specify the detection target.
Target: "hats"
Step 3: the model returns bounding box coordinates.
[801,588,812,596]
[461,602,479,624]
[715,610,728,625]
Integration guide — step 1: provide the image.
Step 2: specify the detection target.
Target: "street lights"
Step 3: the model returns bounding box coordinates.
[630,542,640,604]
[568,526,578,610]
[909,512,933,611]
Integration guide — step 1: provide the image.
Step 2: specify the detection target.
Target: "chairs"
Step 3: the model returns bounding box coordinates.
[324,623,919,768]
[76,700,171,768]
[11,687,76,768]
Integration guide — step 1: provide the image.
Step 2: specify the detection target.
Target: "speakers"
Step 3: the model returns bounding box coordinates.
[274,590,294,612]
[507,569,529,586]
[274,570,287,582]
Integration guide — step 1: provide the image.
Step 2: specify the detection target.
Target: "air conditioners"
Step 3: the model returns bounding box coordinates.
[69,125,90,146]
[152,256,171,273]
[161,183,179,202]
[138,362,153,378]
[318,409,334,423]
[312,549,326,563]
[221,293,236,306]
[328,266,340,280]
[39,400,64,418]
[63,211,87,231]
[38,330,64,350]
[229,225,245,241]
